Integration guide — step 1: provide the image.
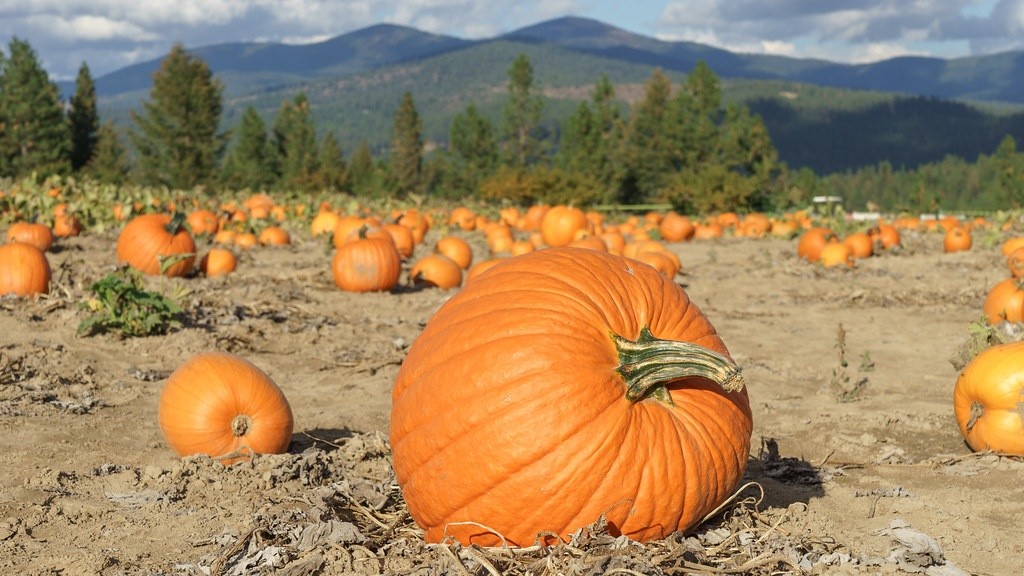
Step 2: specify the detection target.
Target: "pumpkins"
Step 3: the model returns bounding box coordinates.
[0,189,1024,550]
